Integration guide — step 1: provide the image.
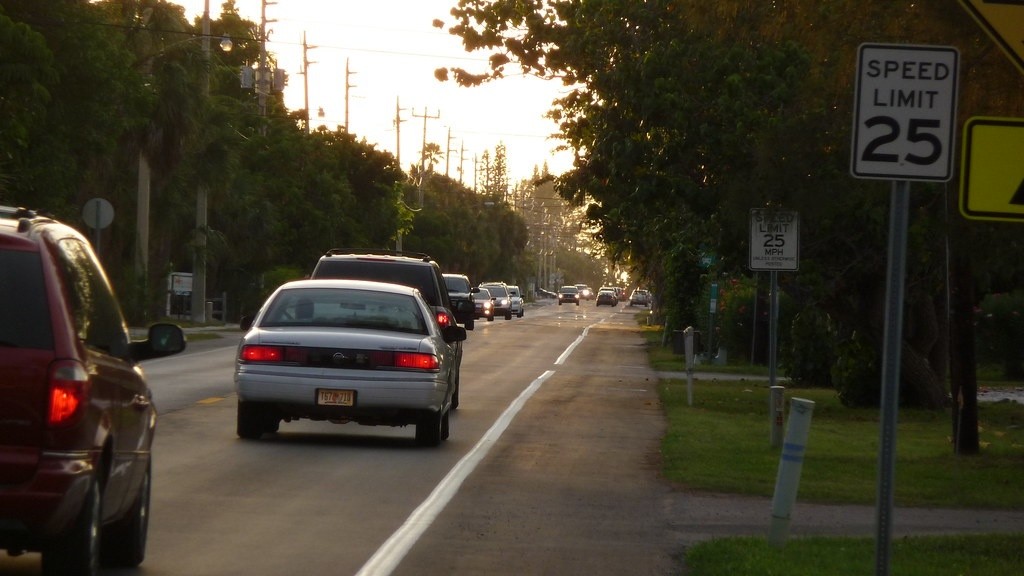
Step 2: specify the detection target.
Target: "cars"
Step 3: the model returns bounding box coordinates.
[233,278,466,447]
[574,282,650,307]
[557,285,580,305]
[471,287,497,321]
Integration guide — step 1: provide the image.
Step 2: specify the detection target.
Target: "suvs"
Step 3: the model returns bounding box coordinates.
[506,284,524,319]
[0,201,189,576]
[477,281,513,320]
[304,249,467,411]
[441,272,480,331]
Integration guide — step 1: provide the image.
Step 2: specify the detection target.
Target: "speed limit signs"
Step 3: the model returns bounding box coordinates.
[749,205,800,272]
[852,44,958,182]
